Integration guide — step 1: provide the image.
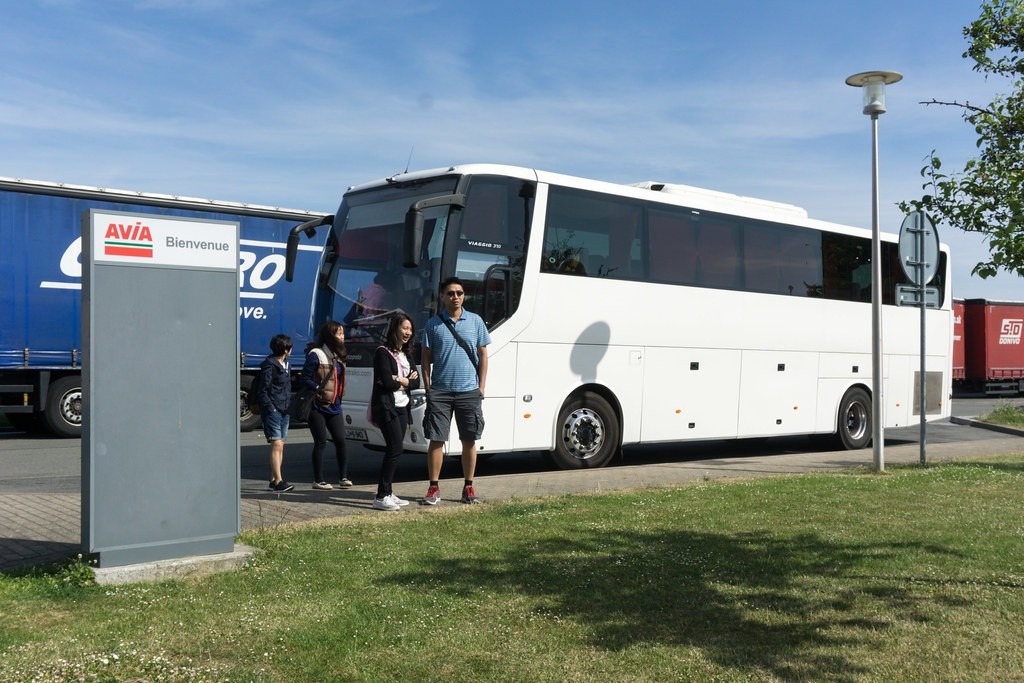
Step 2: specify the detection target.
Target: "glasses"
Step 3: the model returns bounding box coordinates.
[444,291,464,297]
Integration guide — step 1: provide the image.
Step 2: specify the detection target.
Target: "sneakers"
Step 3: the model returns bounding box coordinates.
[373,497,400,510]
[421,486,441,505]
[461,485,479,504]
[273,481,294,492]
[267,480,275,490]
[390,494,410,506]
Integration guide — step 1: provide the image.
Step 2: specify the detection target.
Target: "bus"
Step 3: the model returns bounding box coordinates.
[286,164,953,471]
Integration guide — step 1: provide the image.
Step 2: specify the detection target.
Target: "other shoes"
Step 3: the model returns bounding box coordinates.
[312,481,333,489]
[338,478,353,486]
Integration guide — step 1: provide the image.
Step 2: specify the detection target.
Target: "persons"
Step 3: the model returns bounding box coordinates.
[420,276,491,505]
[301,321,352,490]
[257,334,293,493]
[363,275,387,316]
[371,314,420,510]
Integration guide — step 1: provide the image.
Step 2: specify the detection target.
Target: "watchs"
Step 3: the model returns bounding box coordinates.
[396,376,400,382]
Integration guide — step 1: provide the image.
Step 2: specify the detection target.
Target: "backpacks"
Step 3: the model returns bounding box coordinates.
[246,359,284,415]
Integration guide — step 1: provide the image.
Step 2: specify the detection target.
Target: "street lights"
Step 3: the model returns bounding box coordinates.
[845,69,904,474]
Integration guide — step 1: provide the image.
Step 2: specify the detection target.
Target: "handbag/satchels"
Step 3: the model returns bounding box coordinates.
[286,393,312,421]
[366,398,378,428]
